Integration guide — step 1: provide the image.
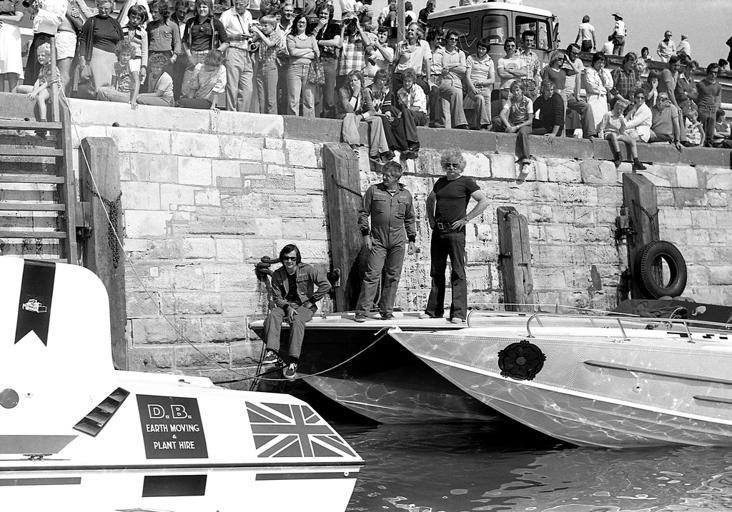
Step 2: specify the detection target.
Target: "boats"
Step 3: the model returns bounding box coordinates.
[249,306,683,425]
[384,327,732,449]
[0,253,367,512]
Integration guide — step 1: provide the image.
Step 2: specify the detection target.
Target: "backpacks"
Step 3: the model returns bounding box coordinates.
[307,51,328,87]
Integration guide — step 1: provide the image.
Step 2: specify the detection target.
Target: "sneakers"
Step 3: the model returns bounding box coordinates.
[452,318,462,323]
[419,314,431,319]
[262,354,277,364]
[353,149,360,158]
[285,354,296,378]
[522,164,530,174]
[355,315,366,322]
[370,156,385,165]
[382,315,395,320]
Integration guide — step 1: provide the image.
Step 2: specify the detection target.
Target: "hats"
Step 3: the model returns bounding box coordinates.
[612,12,623,19]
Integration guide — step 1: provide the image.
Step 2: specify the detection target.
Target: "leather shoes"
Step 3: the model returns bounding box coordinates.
[387,149,395,158]
[615,157,623,165]
[634,163,646,170]
[401,151,415,159]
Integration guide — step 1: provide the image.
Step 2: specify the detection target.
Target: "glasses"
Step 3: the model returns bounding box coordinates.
[435,37,443,40]
[283,256,297,261]
[449,38,459,41]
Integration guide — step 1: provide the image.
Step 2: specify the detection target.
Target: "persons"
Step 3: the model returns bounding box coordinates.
[353,0,372,32]
[624,88,653,143]
[712,110,731,148]
[287,14,320,117]
[97,40,140,108]
[677,34,691,64]
[435,32,467,128]
[249,15,281,115]
[397,72,427,151]
[659,56,696,147]
[636,47,654,83]
[339,1,354,19]
[394,22,446,128]
[15,43,62,133]
[612,12,627,56]
[170,0,193,101]
[532,82,564,137]
[559,43,596,143]
[136,54,173,107]
[144,0,182,101]
[261,244,332,379]
[339,71,389,166]
[576,52,614,140]
[639,71,659,110]
[719,59,727,69]
[177,50,227,113]
[306,3,343,119]
[463,42,495,131]
[56,1,83,97]
[460,0,522,7]
[397,30,469,130]
[695,64,721,147]
[418,0,436,40]
[539,51,567,137]
[406,1,417,38]
[419,149,489,323]
[354,161,417,322]
[23,0,68,86]
[365,69,419,161]
[377,1,396,27]
[77,0,124,98]
[674,59,698,127]
[368,26,394,87]
[657,30,676,63]
[575,16,597,52]
[515,30,542,102]
[648,92,686,149]
[336,12,371,118]
[121,5,149,85]
[0,0,24,93]
[492,81,533,173]
[598,100,647,170]
[274,2,295,115]
[219,0,253,112]
[303,0,333,15]
[610,52,643,117]
[680,110,706,147]
[182,0,230,99]
[497,37,528,117]
[600,36,615,55]
[260,0,281,16]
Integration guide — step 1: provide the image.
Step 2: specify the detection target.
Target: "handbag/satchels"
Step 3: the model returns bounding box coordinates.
[307,51,325,85]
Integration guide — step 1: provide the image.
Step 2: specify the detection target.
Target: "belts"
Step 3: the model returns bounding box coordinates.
[435,222,454,230]
